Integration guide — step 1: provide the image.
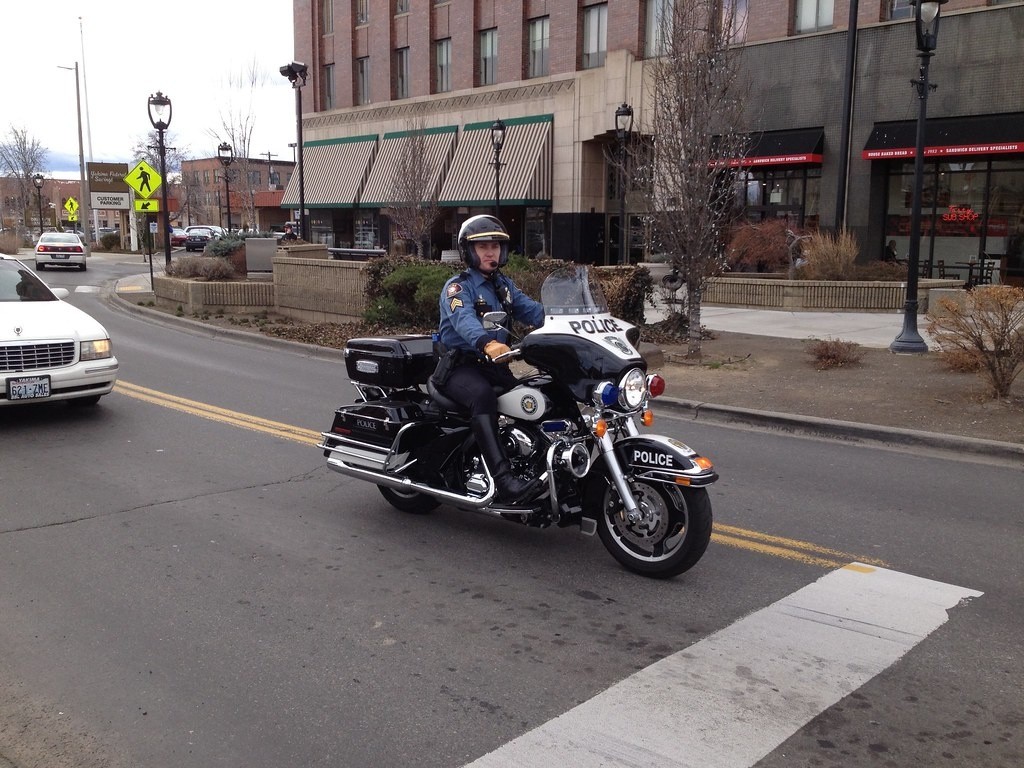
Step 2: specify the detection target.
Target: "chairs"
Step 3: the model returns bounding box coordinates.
[970,262,995,286]
[938,259,960,279]
[918,259,929,278]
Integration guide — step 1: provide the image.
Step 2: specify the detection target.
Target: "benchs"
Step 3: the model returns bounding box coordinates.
[328,247,386,262]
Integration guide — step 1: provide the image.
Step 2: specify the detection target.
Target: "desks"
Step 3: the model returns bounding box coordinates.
[898,259,909,267]
[954,261,981,288]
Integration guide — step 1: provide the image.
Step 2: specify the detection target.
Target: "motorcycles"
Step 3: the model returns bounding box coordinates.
[317,263,720,578]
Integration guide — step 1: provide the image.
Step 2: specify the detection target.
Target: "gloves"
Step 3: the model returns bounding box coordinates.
[484,340,511,363]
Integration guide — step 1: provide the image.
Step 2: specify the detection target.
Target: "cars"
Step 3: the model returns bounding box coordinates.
[34,233,87,271]
[168,223,227,252]
[0,252,120,414]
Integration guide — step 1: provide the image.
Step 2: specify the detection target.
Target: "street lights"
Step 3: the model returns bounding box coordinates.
[148,91,176,270]
[33,174,45,236]
[489,117,507,221]
[889,0,948,353]
[616,103,635,263]
[219,142,235,232]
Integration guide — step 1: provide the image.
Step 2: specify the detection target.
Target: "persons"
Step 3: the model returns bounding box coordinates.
[886,239,902,266]
[281,224,297,242]
[440,214,546,504]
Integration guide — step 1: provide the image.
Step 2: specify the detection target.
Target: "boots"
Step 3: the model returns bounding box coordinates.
[471,415,543,504]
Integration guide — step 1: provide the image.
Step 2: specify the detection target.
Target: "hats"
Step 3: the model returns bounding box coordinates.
[283,224,292,228]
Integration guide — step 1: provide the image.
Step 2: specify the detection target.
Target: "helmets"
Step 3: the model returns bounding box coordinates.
[457,214,511,269]
